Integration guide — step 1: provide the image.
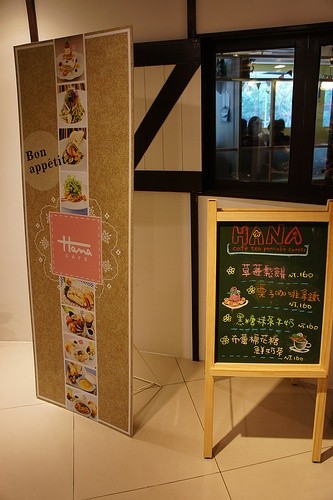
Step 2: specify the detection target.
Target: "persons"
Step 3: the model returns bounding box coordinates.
[242,117,267,169]
[266,120,291,171]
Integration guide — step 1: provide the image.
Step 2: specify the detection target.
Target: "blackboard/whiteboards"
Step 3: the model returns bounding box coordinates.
[201,200,333,378]
